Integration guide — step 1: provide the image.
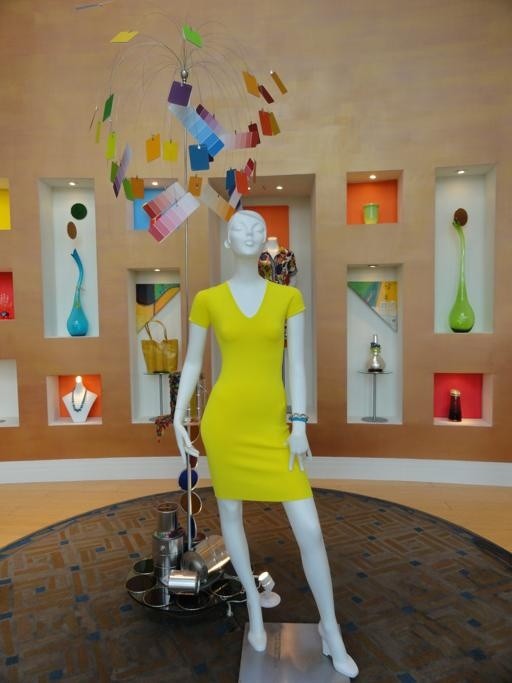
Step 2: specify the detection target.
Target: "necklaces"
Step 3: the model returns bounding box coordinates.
[70,387,89,412]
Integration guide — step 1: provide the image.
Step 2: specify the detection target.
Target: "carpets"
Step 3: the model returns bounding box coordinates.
[1,483,512,682]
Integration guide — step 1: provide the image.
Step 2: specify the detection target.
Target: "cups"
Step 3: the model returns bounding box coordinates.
[363,203,379,224]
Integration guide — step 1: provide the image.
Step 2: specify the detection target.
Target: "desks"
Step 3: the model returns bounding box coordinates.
[357,369,392,422]
[144,372,172,423]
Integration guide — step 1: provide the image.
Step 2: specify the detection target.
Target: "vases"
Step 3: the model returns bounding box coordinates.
[449,218,476,333]
[361,202,380,224]
[66,247,89,336]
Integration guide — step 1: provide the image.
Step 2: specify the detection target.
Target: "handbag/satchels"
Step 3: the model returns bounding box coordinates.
[141,321,179,373]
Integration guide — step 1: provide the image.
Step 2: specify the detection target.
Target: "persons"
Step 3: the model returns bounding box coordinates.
[60,374,99,424]
[173,209,362,679]
[256,234,298,348]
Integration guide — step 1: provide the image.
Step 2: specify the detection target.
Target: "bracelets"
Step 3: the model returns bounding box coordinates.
[287,409,311,423]
